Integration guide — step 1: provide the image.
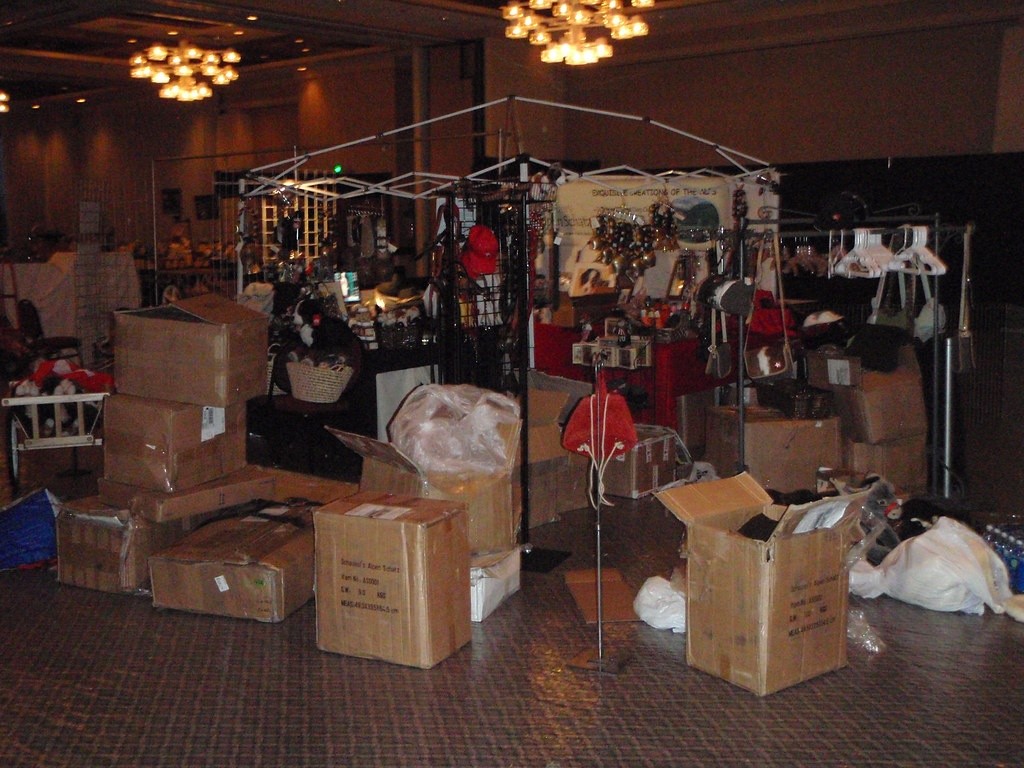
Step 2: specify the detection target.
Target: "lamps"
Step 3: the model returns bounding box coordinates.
[129,40,241,101]
[501,0,654,65]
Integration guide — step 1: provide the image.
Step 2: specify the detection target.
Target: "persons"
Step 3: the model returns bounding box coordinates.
[883,499,985,541]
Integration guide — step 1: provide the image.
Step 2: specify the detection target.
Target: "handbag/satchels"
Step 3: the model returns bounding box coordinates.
[914,298,948,342]
[709,343,733,379]
[697,272,797,336]
[875,308,908,331]
[387,382,521,474]
[847,516,1014,615]
[844,322,911,373]
[742,340,793,381]
[943,327,976,373]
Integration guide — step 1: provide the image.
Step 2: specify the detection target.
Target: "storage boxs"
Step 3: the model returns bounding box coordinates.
[53,293,928,697]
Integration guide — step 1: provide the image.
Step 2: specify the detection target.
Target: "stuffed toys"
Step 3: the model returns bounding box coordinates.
[287,299,363,370]
[8,359,113,438]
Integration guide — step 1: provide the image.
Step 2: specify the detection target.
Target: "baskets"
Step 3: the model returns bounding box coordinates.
[286,352,354,403]
[259,352,287,395]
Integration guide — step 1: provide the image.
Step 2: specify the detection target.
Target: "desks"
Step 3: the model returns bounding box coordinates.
[532,311,799,459]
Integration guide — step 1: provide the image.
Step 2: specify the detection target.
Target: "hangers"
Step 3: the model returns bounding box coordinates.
[782,224,946,278]
